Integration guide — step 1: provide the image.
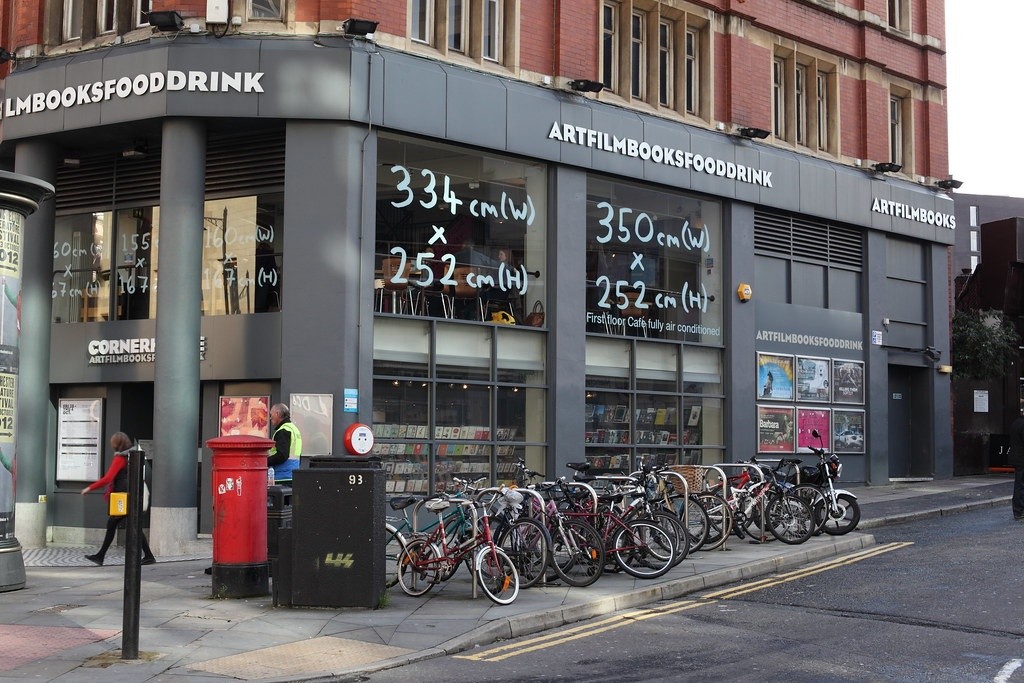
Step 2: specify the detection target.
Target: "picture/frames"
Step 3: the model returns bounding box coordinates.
[755,351,866,406]
[755,404,866,454]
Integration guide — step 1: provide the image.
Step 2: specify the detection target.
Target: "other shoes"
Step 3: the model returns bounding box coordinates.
[85,554,104,566]
[140,558,156,565]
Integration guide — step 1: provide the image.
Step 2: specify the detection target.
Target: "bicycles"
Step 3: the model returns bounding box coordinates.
[383,455,831,607]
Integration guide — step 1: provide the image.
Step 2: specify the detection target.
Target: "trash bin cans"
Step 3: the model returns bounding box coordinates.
[265,486,293,577]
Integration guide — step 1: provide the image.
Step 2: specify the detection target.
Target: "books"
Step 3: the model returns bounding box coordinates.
[585,403,702,481]
[372,424,518,492]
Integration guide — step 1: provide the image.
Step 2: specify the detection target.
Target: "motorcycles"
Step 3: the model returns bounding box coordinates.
[745,429,861,536]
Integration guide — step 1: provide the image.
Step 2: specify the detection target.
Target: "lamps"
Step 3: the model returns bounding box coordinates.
[570,79,605,93]
[123,137,150,157]
[341,18,380,36]
[873,162,902,173]
[741,127,771,139]
[145,10,184,31]
[64,145,81,164]
[936,179,963,189]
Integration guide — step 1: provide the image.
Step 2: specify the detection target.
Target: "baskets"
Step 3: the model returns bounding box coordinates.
[668,465,704,494]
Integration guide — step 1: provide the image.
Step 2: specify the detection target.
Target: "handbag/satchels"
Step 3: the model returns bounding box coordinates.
[523,300,544,327]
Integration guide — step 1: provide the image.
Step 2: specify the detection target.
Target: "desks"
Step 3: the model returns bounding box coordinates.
[610,301,652,338]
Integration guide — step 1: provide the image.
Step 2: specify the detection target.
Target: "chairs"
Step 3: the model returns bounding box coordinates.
[375,259,516,325]
[586,286,648,338]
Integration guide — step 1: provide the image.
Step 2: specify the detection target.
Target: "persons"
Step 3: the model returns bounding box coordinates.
[460,239,519,300]
[80,432,157,565]
[1008,418,1024,518]
[267,403,302,487]
[497,248,514,271]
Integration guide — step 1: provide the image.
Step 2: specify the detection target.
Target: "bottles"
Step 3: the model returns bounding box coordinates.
[267,466,275,486]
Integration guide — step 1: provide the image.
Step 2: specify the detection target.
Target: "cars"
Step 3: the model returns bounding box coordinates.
[834,429,863,449]
